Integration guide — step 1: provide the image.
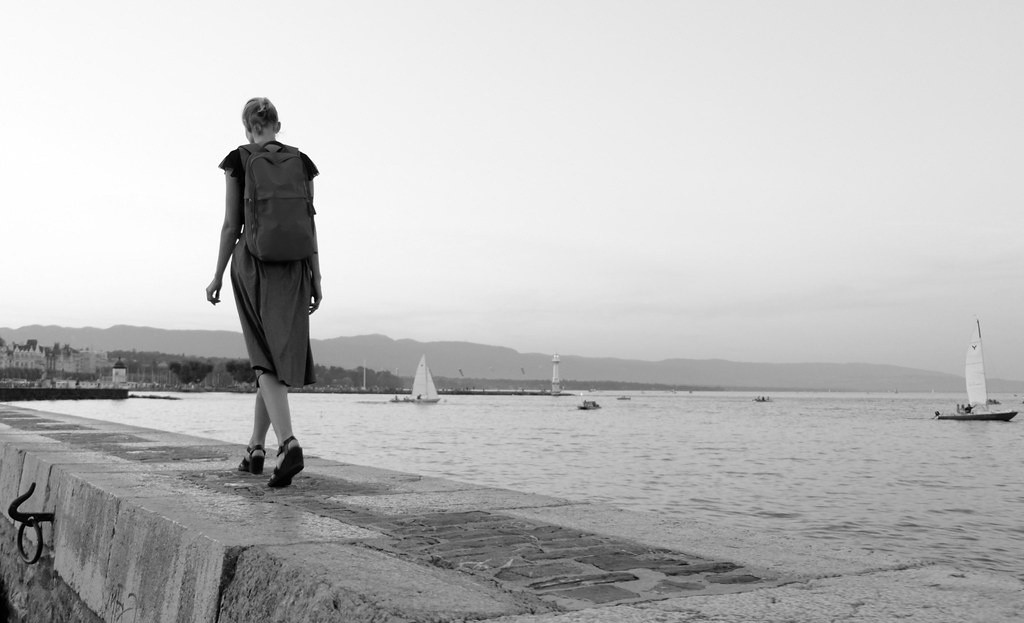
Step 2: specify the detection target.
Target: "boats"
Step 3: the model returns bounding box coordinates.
[577,400,602,411]
[389,396,414,402]
[752,396,773,402]
[616,396,631,400]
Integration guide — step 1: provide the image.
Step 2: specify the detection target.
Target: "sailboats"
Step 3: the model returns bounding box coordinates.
[408,354,442,403]
[934,317,1019,421]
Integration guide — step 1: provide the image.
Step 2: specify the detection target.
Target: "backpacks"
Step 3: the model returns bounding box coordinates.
[239,142,316,261]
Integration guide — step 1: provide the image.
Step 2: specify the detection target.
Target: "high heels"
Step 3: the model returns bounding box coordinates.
[268,437,305,487]
[239,445,266,474]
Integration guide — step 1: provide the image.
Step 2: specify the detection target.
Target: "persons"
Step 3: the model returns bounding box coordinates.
[205,97,324,486]
[957,403,977,414]
